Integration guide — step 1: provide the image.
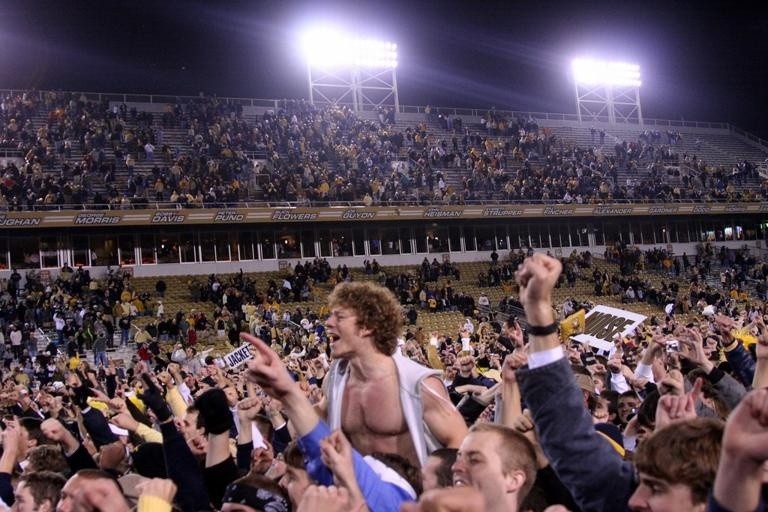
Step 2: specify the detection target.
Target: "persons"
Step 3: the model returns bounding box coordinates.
[1,87,768,208]
[0,237,768,512]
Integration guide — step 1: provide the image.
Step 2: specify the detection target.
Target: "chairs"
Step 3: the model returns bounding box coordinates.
[454,253,702,337]
[135,269,469,377]
